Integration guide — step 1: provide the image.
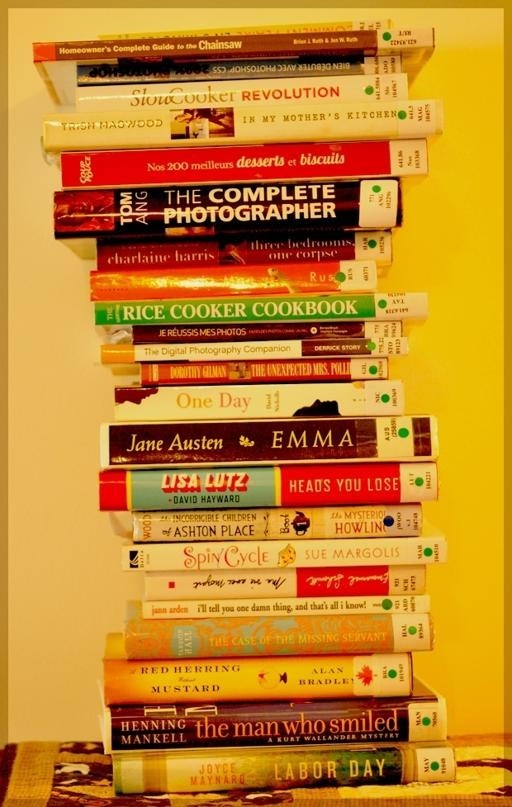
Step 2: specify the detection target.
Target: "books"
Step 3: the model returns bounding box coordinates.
[31,17,460,796]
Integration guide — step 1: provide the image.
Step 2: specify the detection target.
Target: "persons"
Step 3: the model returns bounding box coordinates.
[174,108,231,131]
